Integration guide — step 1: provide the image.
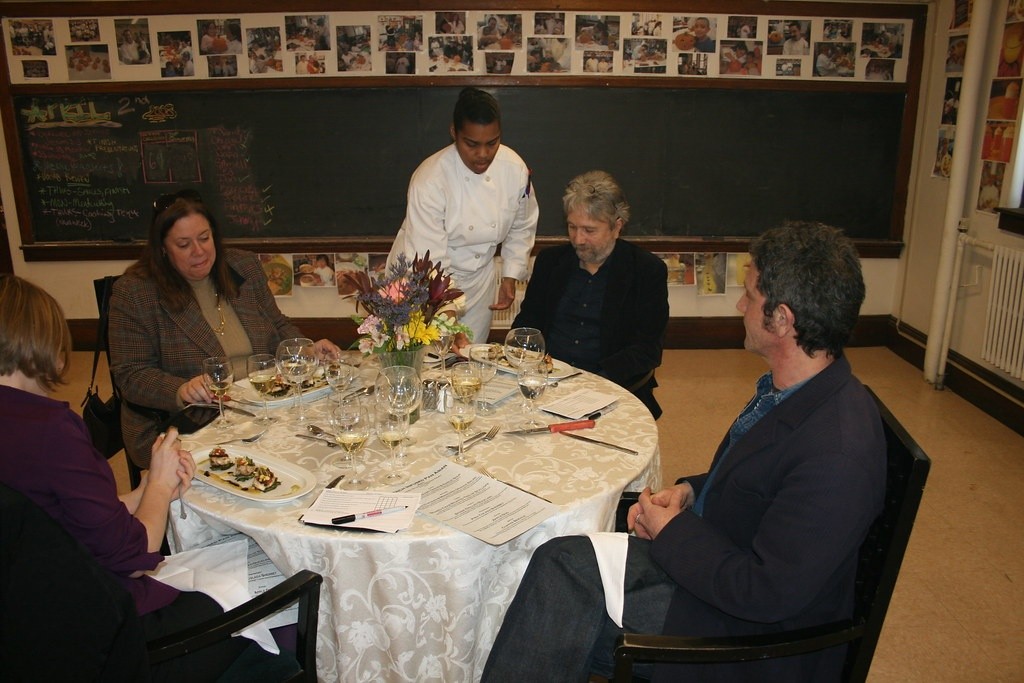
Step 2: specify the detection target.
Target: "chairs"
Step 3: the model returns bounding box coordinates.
[0,482,323,683]
[615,385,933,683]
[93,274,143,491]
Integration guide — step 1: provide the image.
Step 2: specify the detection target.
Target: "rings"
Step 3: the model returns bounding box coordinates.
[176,438,181,441]
[635,514,640,523]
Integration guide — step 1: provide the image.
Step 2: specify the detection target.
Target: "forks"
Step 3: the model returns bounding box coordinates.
[295,434,339,447]
[479,468,552,503]
[548,372,583,388]
[454,424,501,456]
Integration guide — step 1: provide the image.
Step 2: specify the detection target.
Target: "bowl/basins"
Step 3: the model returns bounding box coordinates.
[300,275,316,287]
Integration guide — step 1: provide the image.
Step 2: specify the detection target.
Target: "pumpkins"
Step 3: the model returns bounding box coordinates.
[213,35,227,52]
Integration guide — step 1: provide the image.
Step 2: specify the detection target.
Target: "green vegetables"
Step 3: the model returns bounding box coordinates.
[210,457,282,493]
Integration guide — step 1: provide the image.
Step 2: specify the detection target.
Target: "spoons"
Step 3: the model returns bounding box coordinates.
[307,425,335,438]
[446,431,487,452]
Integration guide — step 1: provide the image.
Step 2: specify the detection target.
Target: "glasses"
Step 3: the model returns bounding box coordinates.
[153,188,202,223]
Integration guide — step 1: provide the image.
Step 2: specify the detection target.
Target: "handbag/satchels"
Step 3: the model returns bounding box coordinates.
[82,393,125,460]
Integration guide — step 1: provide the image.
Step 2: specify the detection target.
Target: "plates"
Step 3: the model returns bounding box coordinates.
[299,264,314,273]
[188,444,317,504]
[231,361,362,413]
[459,343,576,380]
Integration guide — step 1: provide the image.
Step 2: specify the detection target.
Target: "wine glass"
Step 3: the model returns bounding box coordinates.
[469,345,498,417]
[374,365,423,446]
[431,335,455,384]
[248,354,277,428]
[324,350,354,420]
[326,390,362,469]
[450,362,483,437]
[517,362,548,428]
[374,401,410,485]
[504,327,546,415]
[274,337,318,431]
[445,393,477,467]
[202,356,234,431]
[332,404,371,491]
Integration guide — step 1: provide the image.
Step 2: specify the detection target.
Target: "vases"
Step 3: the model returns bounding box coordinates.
[376,346,427,425]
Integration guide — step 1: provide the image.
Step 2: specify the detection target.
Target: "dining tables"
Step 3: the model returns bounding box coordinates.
[161,344,662,683]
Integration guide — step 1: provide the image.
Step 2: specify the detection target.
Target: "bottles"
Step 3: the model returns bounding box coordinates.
[436,381,452,413]
[423,379,437,412]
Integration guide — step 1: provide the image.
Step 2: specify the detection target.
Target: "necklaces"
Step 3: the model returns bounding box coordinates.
[209,293,226,335]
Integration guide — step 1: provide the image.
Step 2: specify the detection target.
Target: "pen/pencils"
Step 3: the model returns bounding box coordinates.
[425,351,439,359]
[587,404,619,421]
[330,504,410,526]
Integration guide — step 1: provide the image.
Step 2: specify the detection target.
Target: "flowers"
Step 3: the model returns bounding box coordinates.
[341,249,474,359]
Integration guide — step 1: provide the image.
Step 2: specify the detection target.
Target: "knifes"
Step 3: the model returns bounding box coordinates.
[500,420,595,436]
[298,474,345,525]
[559,431,639,456]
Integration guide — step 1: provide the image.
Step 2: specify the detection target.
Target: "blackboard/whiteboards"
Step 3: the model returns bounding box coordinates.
[1,79,920,258]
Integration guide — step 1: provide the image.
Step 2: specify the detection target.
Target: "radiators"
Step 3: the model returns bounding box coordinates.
[981,245,1024,382]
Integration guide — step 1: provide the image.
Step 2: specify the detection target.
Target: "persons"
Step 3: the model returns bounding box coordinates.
[247,28,279,73]
[383,85,540,355]
[383,11,521,74]
[107,190,341,472]
[70,19,97,40]
[200,21,242,77]
[0,275,300,683]
[527,13,619,74]
[623,13,809,76]
[312,255,335,286]
[9,22,55,55]
[160,39,194,77]
[511,169,669,422]
[119,29,149,64]
[812,20,904,81]
[931,0,1000,212]
[287,18,369,75]
[478,219,888,683]
